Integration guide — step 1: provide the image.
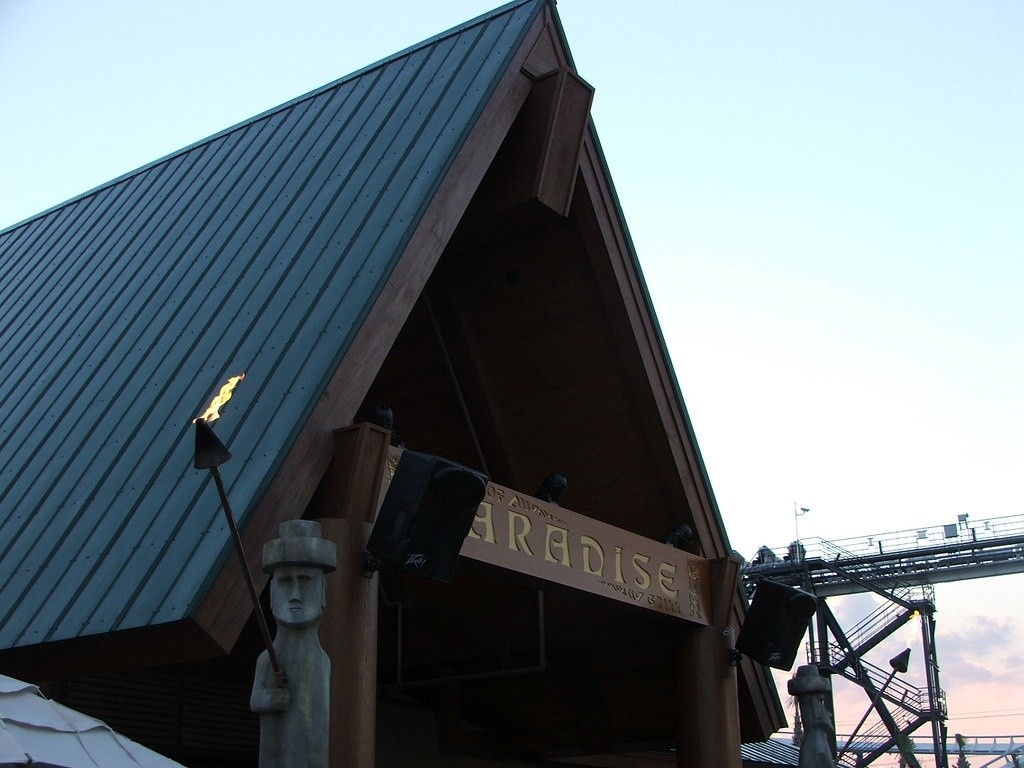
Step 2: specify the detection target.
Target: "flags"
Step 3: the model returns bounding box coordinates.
[735,579,818,672]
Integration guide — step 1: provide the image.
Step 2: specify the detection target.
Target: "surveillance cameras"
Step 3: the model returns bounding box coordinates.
[801,508,809,512]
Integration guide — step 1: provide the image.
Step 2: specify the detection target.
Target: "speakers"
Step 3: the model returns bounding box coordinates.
[365,448,490,585]
[734,580,818,671]
